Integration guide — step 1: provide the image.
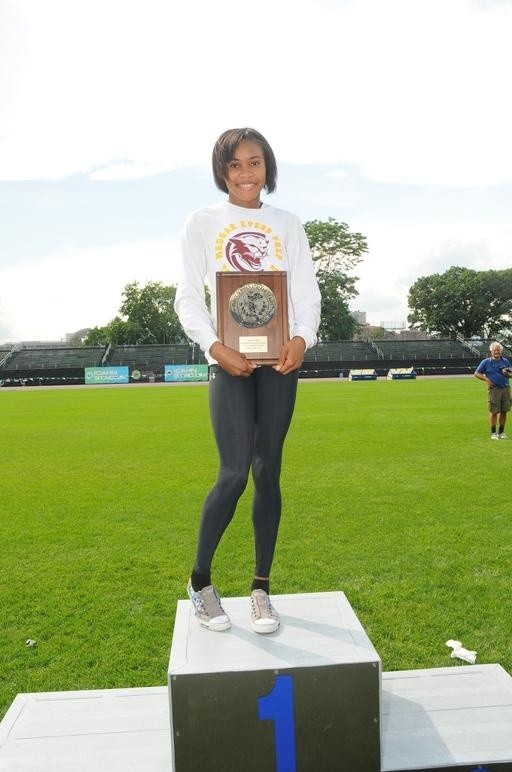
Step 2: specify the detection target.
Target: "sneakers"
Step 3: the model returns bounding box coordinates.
[491,432,508,440]
[249,589,280,633]
[188,577,232,632]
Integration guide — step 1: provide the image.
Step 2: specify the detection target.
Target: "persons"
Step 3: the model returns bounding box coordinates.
[474,341,512,440]
[172,128,322,635]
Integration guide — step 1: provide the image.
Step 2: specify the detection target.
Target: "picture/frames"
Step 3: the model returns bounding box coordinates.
[216,271,289,365]
